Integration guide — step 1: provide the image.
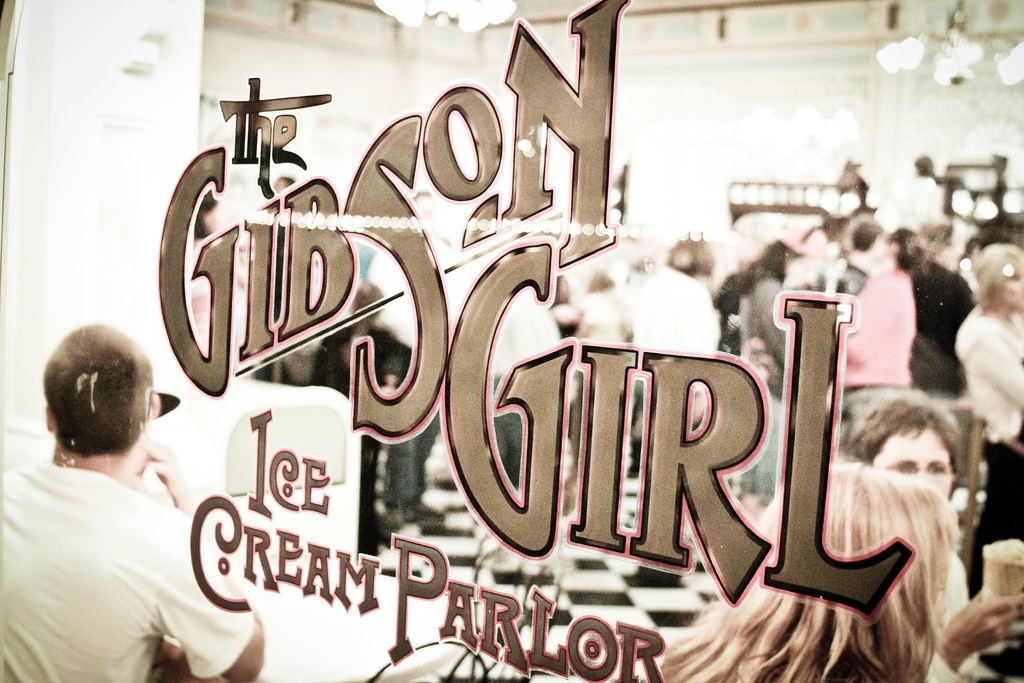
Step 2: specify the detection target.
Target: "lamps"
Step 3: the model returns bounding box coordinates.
[876,0,1024,87]
[374,0,518,33]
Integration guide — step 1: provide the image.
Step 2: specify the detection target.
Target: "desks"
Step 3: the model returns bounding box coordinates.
[163,564,497,683]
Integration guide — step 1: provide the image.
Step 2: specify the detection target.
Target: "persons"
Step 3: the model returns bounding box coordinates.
[190,157,1024,682]
[0,323,265,683]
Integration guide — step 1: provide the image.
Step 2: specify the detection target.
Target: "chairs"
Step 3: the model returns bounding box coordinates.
[853,407,987,597]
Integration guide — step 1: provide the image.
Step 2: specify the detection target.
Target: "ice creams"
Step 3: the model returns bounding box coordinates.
[980,538,1024,597]
